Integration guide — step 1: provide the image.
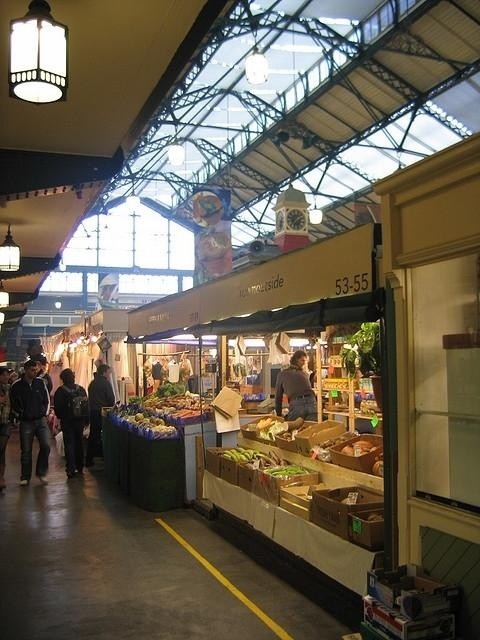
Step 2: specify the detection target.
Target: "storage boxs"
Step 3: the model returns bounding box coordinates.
[242,399,267,414]
[206,411,386,552]
[359,593,461,640]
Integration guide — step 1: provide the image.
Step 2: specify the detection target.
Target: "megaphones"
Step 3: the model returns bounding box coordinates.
[249,239,267,256]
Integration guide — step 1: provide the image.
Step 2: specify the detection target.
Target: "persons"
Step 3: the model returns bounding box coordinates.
[0,367,14,488]
[84,363,115,468]
[38,367,53,395]
[274,366,318,422]
[55,368,90,479]
[290,350,307,369]
[9,370,20,427]
[11,361,54,487]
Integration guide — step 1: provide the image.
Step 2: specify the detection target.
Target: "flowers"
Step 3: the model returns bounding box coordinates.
[339,318,382,376]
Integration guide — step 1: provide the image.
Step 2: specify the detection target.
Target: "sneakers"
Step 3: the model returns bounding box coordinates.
[40,476,48,484]
[20,480,29,485]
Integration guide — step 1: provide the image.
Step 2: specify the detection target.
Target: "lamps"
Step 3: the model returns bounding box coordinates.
[0,282,10,308]
[54,296,62,311]
[168,123,187,168]
[242,25,269,84]
[307,192,324,226]
[59,254,67,272]
[0,218,23,273]
[124,178,142,216]
[6,0,73,106]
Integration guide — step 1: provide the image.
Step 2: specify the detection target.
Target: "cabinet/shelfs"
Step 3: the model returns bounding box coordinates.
[349,362,383,438]
[314,340,354,433]
[100,403,220,514]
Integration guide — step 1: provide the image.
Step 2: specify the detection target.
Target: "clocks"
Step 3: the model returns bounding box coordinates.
[274,208,286,235]
[285,205,308,234]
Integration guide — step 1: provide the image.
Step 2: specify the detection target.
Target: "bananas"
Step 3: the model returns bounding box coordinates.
[223,447,265,462]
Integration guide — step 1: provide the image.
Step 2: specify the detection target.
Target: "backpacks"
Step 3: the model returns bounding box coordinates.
[60,384,89,417]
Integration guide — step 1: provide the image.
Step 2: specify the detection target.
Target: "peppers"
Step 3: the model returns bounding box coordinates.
[268,466,310,477]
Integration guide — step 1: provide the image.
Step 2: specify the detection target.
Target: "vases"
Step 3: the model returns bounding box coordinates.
[369,372,383,409]
